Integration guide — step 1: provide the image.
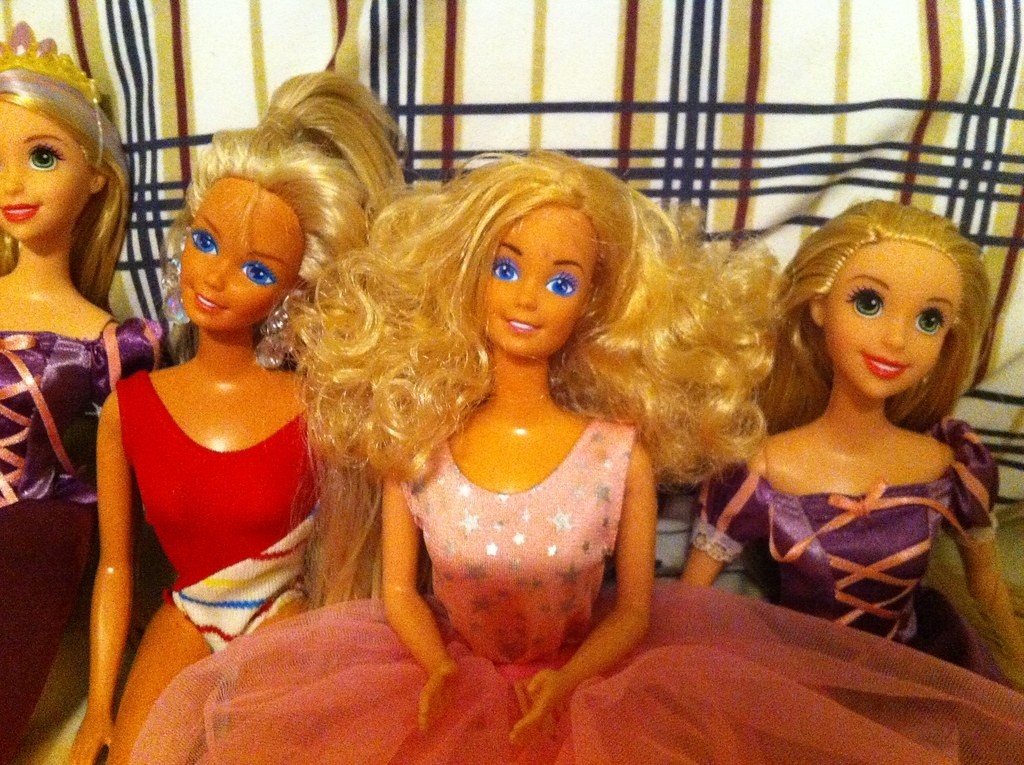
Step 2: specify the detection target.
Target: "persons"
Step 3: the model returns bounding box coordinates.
[683,198,1024,691]
[0,25,1024,765]
